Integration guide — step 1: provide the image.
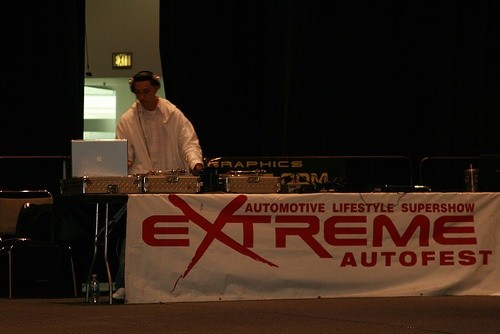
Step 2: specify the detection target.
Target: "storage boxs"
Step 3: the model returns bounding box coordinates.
[60,170,204,196]
[218,170,282,194]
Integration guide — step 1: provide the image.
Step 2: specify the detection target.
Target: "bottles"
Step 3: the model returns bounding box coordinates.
[88,274,100,305]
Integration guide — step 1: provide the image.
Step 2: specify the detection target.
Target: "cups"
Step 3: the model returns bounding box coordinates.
[462,157,483,193]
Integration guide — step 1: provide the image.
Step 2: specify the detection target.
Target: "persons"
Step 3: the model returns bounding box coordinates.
[112,71,204,301]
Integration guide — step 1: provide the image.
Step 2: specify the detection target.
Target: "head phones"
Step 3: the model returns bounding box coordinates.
[129,70,160,93]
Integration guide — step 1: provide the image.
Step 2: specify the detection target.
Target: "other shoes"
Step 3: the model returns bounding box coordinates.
[111,287,125,300]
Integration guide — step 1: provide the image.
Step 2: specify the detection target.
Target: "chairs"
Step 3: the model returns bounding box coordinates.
[0,189,78,299]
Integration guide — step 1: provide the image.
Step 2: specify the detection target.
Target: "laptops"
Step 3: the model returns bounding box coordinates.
[71,139,128,178]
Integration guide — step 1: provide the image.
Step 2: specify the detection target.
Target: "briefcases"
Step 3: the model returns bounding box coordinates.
[59,173,143,196]
[222,168,282,193]
[143,168,202,194]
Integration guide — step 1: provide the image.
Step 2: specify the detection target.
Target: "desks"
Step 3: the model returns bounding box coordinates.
[62,188,500,305]
